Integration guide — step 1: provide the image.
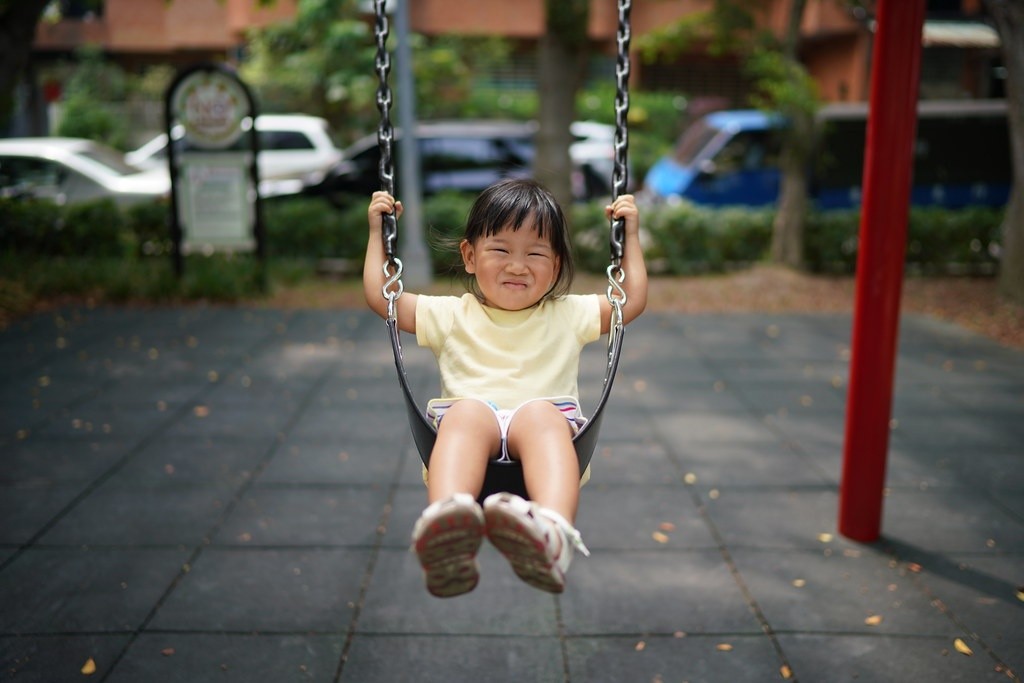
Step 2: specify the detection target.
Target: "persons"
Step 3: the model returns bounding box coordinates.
[363,178,649,598]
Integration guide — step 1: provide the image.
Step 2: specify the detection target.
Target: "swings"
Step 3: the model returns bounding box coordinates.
[373,0,631,508]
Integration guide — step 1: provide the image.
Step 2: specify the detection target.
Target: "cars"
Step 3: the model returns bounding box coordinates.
[296,122,635,204]
[0,134,163,204]
[120,113,339,196]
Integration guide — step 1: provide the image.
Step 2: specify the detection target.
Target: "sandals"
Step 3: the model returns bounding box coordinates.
[412,492,483,598]
[483,493,590,594]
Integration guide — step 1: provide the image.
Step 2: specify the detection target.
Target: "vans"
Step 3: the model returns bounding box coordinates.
[633,102,1014,216]
